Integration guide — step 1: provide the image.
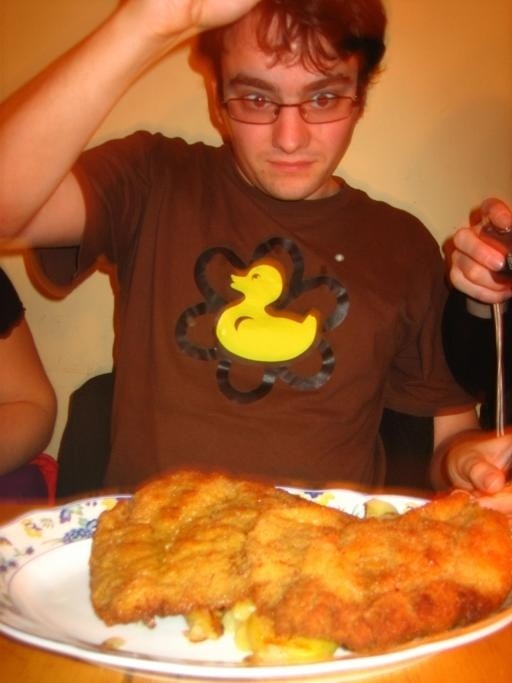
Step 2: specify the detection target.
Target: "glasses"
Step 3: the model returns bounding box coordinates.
[220,96,361,125]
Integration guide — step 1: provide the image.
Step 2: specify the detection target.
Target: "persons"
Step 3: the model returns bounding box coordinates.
[440,197,511,437]
[0,262,59,474]
[0,0,511,499]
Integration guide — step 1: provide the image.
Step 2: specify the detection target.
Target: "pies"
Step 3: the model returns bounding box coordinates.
[92,472,512,652]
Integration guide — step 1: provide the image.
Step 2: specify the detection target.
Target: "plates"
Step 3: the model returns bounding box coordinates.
[0,487,510,677]
